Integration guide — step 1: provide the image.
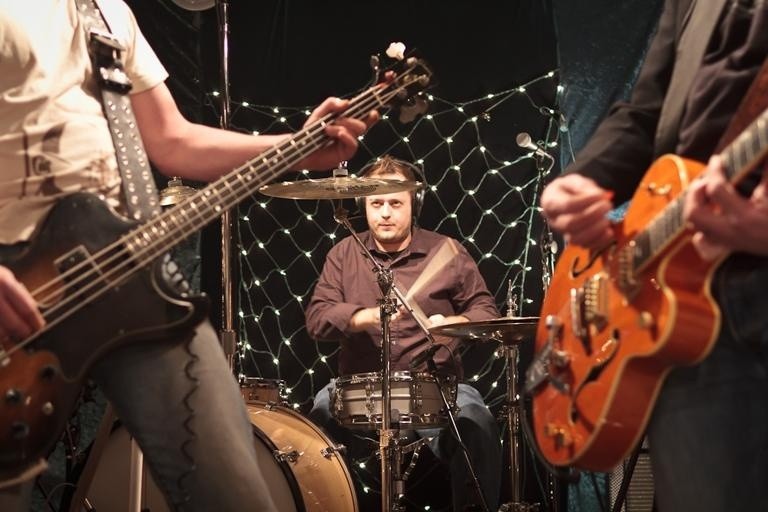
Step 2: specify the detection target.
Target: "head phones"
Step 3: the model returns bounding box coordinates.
[353,156,430,219]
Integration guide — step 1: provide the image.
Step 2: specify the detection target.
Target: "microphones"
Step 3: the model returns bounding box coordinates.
[515,132,551,162]
[407,344,442,368]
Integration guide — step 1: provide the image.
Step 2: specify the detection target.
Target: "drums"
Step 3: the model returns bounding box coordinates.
[328,371,460,429]
[237,371,289,409]
[70,400,359,512]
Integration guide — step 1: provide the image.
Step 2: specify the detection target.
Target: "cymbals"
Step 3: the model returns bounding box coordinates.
[158,187,198,206]
[428,317,538,343]
[258,176,426,199]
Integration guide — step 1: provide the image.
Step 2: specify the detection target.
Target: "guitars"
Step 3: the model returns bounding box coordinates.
[0,42,432,488]
[526,109,768,470]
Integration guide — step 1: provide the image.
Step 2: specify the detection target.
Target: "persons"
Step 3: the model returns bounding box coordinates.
[540,1,767,512]
[305,157,507,512]
[0,0,380,511]
